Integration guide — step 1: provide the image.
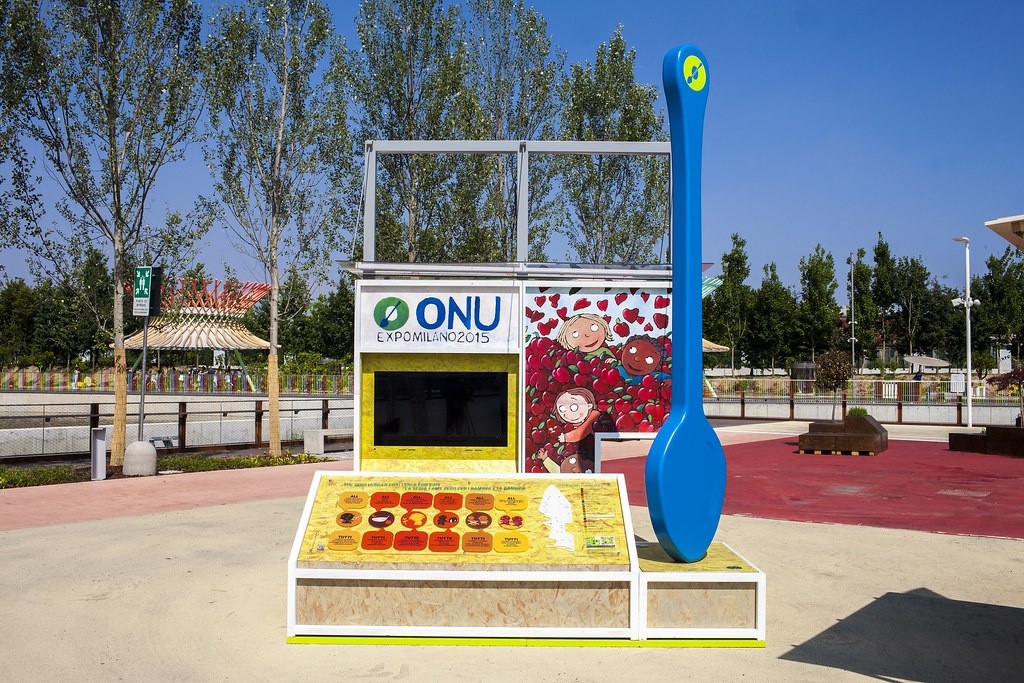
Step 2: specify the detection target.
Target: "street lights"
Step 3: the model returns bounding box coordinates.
[846,252,858,367]
[952,236,980,429]
[1017,343,1023,369]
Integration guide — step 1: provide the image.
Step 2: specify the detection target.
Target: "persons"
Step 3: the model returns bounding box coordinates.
[73,367,80,390]
[145,365,242,390]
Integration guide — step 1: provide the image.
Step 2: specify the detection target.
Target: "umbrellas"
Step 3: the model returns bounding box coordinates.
[703,338,730,351]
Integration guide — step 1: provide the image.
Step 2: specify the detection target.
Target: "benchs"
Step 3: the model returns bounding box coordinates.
[303,428,354,454]
